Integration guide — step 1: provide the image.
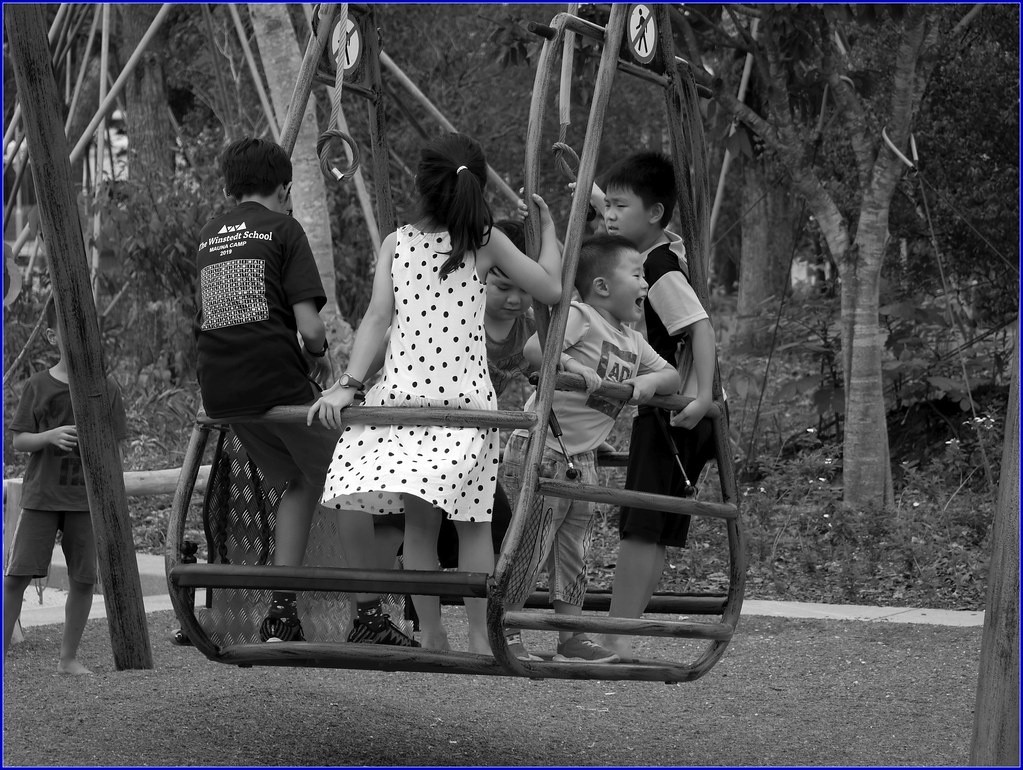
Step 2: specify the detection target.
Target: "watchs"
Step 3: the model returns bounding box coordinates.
[304,338,328,357]
[338,374,364,390]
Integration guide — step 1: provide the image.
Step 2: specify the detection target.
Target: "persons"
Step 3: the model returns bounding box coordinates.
[306,131,562,654]
[196,138,421,648]
[343,220,538,639]
[569,153,717,662]
[501,233,679,663]
[5,303,127,673]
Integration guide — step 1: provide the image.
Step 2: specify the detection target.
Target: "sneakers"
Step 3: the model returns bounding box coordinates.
[502,625,531,662]
[551,633,621,663]
[260,611,306,643]
[346,614,421,647]
